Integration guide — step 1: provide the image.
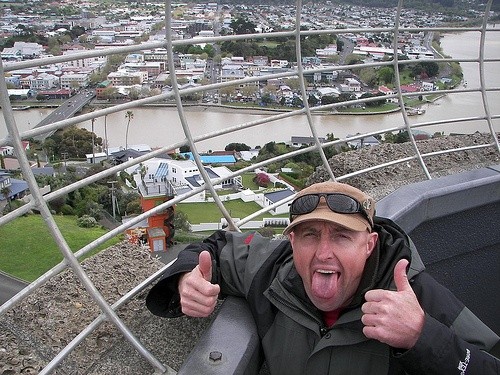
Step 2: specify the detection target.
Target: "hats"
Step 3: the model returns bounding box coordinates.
[282,181,377,232]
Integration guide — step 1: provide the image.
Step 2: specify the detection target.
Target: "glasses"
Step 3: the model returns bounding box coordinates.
[289,192,374,228]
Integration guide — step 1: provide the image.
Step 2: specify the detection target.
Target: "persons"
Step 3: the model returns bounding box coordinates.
[143,183,500,375]
